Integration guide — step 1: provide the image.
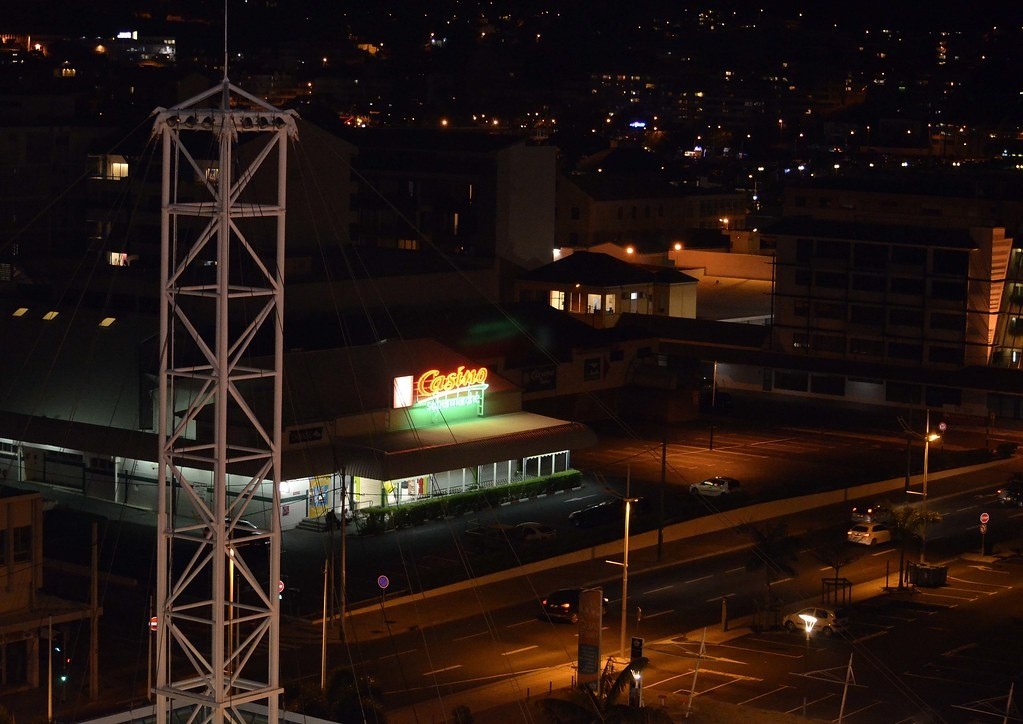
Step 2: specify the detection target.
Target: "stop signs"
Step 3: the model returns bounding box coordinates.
[980,513,990,524]
[149,616,158,630]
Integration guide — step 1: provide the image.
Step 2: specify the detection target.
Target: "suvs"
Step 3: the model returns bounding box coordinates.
[781,607,852,637]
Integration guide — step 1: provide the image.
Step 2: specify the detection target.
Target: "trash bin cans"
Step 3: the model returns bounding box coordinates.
[915,563,947,586]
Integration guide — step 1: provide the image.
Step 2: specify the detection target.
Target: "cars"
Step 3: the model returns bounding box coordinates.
[512,523,557,544]
[995,485,1023,508]
[538,587,610,624]
[688,477,731,498]
[850,502,896,525]
[848,522,892,550]
[201,517,272,548]
[567,499,638,524]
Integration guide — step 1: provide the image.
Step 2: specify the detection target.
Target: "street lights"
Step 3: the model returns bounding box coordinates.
[797,613,821,717]
[894,407,942,566]
[592,461,643,663]
